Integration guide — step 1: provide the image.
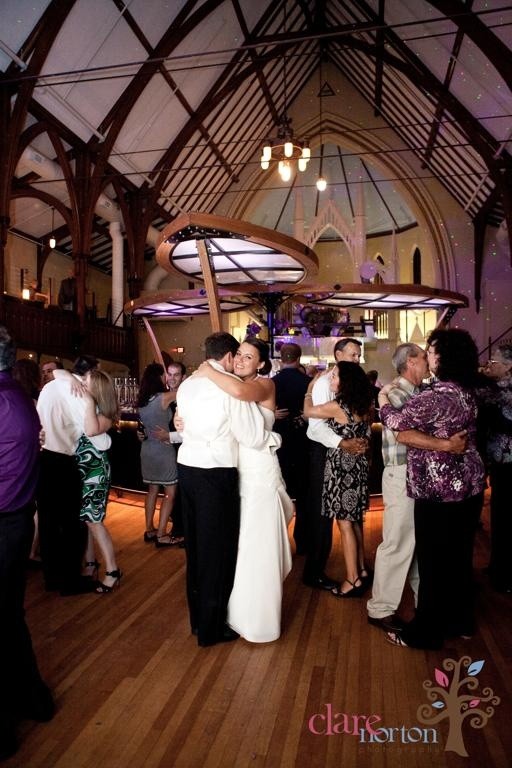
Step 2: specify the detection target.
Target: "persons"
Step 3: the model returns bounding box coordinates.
[300,337,375,592]
[0,323,60,766]
[270,342,315,557]
[478,341,511,597]
[375,322,486,653]
[364,341,473,635]
[8,355,510,599]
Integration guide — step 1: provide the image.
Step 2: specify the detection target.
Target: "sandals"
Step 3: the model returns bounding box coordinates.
[144,529,158,542]
[155,534,178,548]
[384,631,412,649]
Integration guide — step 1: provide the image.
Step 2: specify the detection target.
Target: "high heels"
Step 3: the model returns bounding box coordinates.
[359,568,370,584]
[95,567,123,593]
[332,577,367,600]
[83,560,101,578]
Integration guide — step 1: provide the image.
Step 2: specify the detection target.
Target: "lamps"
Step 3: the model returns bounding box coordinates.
[49,207,56,248]
[260,0,327,192]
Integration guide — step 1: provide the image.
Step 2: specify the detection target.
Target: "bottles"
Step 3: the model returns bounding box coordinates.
[110,375,139,405]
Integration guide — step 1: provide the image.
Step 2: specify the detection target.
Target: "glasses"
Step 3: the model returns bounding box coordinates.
[486,359,503,369]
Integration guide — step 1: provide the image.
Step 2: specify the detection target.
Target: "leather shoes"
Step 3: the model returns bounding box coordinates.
[367,613,407,633]
[197,628,240,647]
[304,576,340,590]
